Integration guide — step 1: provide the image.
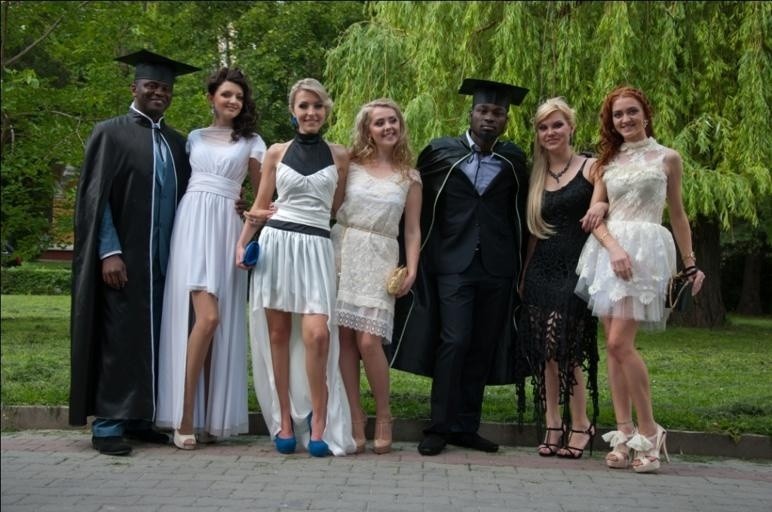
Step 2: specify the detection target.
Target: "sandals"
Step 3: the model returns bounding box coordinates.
[197,428,216,444]
[173,426,196,450]
[351,409,368,453]
[373,414,393,455]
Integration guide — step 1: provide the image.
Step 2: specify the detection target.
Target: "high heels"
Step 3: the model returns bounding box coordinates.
[631,424,670,472]
[601,425,637,468]
[538,418,568,457]
[556,424,597,458]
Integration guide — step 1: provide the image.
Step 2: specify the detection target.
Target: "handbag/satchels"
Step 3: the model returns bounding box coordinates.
[387,265,407,295]
[242,240,259,266]
[664,266,703,313]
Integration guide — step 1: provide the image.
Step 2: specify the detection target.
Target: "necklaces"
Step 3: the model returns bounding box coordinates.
[544,153,574,184]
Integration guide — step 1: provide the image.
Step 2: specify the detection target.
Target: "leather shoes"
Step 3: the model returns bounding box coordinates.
[92,437,131,455]
[418,434,448,455]
[122,430,169,444]
[276,415,296,454]
[308,413,329,457]
[469,435,498,452]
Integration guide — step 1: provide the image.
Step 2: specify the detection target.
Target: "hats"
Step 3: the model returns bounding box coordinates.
[457,78,531,130]
[113,49,201,82]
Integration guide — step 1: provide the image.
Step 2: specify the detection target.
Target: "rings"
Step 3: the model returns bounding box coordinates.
[251,218,257,224]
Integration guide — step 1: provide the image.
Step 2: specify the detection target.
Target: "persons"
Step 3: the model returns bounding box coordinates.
[232,77,360,457]
[571,85,708,473]
[515,97,608,460]
[153,69,272,450]
[268,98,423,453]
[382,95,534,457]
[68,69,190,456]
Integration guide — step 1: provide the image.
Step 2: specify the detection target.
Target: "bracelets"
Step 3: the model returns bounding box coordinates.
[680,250,697,263]
[681,265,699,277]
[597,231,611,245]
